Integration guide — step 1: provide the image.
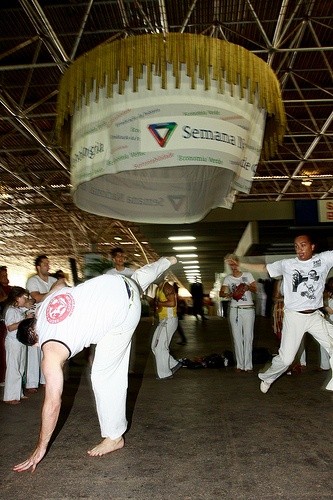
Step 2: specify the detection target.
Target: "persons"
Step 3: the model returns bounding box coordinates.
[220,254,333,374]
[0,241,189,405]
[13,257,178,473]
[227,234,333,393]
[190,276,206,323]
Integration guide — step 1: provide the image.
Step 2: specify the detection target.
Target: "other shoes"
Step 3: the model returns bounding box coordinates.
[0,382,5,387]
[178,357,191,368]
[195,317,208,319]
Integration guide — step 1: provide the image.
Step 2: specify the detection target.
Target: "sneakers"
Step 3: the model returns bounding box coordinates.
[155,363,182,379]
[260,380,271,393]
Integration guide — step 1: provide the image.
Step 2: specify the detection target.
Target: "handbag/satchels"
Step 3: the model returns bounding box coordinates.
[251,347,271,364]
[189,350,234,369]
